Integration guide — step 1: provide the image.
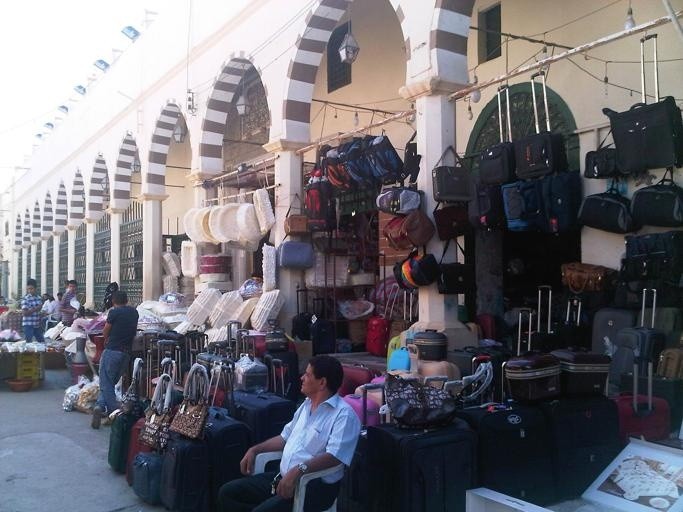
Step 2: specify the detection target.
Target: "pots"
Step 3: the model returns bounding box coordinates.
[411,327,448,361]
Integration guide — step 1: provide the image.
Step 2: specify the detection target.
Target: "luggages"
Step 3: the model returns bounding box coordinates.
[108,320,296,512]
[603,35,683,174]
[478,71,564,187]
[292,289,335,355]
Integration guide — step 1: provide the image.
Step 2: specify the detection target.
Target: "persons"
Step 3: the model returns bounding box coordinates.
[215,354,362,512]
[0,278,79,343]
[90,290,139,429]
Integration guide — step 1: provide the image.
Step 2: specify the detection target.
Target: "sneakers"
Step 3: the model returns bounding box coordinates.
[91,406,102,429]
[101,409,125,425]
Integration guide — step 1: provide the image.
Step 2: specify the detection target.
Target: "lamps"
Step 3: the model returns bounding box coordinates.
[173,117,186,143]
[336,3,359,65]
[235,64,251,115]
[130,146,140,173]
[100,171,109,194]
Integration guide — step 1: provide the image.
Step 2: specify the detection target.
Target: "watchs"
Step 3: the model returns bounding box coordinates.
[297,462,311,475]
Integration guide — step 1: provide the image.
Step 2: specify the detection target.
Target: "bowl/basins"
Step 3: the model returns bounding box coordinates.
[5,378,32,392]
[347,273,377,285]
[88,333,103,344]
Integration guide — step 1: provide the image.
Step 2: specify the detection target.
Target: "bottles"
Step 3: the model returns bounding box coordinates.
[72,336,87,365]
[91,335,104,363]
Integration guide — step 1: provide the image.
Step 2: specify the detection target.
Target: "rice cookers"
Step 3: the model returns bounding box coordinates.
[264,326,288,351]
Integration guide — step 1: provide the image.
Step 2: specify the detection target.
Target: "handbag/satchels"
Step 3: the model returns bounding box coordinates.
[478,175,580,233]
[393,253,438,289]
[437,262,475,294]
[434,206,470,240]
[576,192,642,234]
[285,214,311,235]
[585,148,629,178]
[375,187,436,252]
[432,166,472,204]
[277,242,314,269]
[304,135,408,274]
[561,230,683,309]
[629,184,683,227]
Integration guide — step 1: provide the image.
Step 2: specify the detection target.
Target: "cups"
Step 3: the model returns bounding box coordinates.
[6,340,55,352]
